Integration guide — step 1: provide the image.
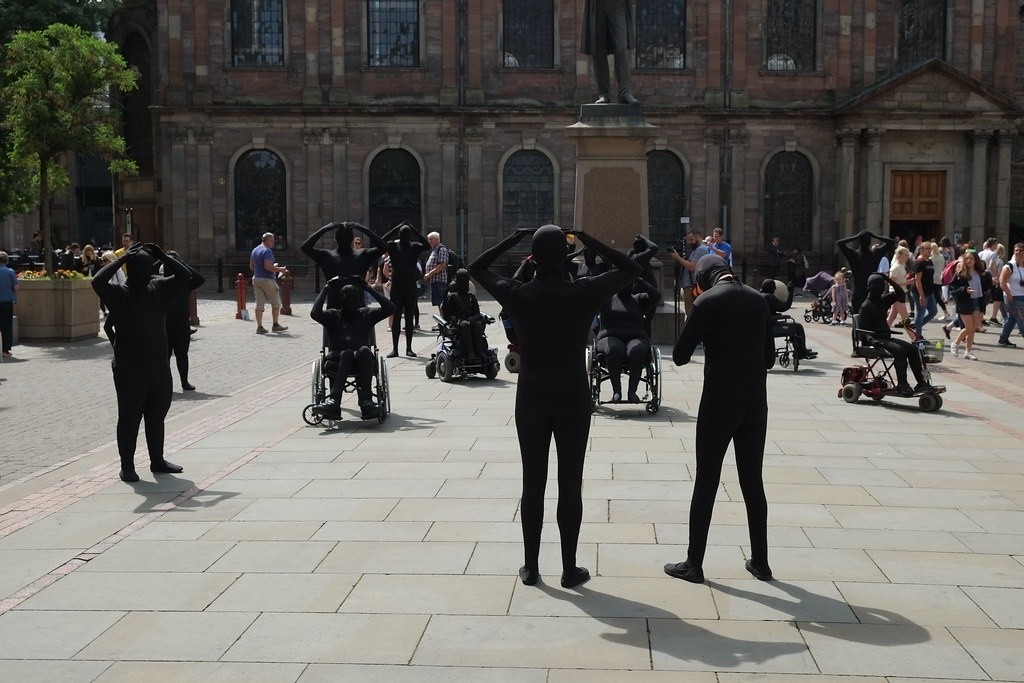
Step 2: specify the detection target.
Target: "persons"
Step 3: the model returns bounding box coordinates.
[702,227,732,270]
[0,251,20,357]
[663,254,776,582]
[858,272,934,396]
[423,231,449,330]
[670,229,708,349]
[768,236,784,273]
[870,235,1024,347]
[250,232,288,334]
[300,219,389,407]
[759,279,818,360]
[381,218,433,358]
[499,232,662,404]
[836,230,896,358]
[90,242,192,482]
[947,249,993,361]
[354,236,424,332]
[788,246,806,297]
[829,272,849,327]
[442,268,488,359]
[310,275,397,420]
[31,230,206,390]
[465,224,644,588]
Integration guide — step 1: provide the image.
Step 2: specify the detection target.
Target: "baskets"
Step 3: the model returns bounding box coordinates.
[923,339,944,363]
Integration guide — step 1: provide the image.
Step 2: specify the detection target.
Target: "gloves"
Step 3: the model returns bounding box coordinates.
[503,223,607,259]
[117,242,168,268]
[323,221,345,233]
[350,274,370,291]
[395,218,415,232]
[326,276,348,288]
[347,221,369,234]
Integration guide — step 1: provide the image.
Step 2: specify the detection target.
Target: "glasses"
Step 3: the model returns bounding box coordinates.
[101,259,107,264]
[355,242,361,245]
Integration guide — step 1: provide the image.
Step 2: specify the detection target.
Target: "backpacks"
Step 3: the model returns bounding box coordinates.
[940,260,964,285]
[803,254,809,268]
[437,246,463,278]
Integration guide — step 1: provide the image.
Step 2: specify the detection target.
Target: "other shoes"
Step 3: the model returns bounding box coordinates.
[183,384,195,390]
[406,351,417,357]
[313,400,342,419]
[612,394,641,403]
[794,293,803,298]
[897,381,934,396]
[182,383,195,390]
[119,471,139,481]
[150,460,183,473]
[272,325,288,331]
[746,559,773,581]
[560,567,590,587]
[663,562,705,583]
[257,327,268,333]
[894,312,1017,361]
[519,566,538,585]
[829,320,847,326]
[191,329,197,334]
[361,400,384,418]
[387,351,398,357]
[4,352,12,357]
[792,351,818,359]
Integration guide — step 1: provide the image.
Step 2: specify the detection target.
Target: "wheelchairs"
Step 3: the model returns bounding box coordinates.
[585,311,663,414]
[425,285,501,382]
[837,314,947,412]
[769,312,816,373]
[301,325,392,431]
[499,308,518,373]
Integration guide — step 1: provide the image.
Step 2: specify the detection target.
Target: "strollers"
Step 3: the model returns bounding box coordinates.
[802,267,852,324]
